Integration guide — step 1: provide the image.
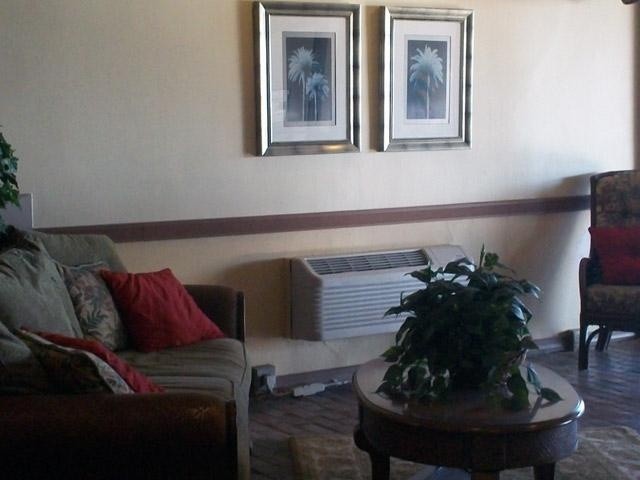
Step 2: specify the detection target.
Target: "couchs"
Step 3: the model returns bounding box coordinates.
[1,222,255,479]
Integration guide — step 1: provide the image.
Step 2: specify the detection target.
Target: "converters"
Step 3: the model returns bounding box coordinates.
[292,382,326,398]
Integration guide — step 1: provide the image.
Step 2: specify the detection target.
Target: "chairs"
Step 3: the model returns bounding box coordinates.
[576,168,639,373]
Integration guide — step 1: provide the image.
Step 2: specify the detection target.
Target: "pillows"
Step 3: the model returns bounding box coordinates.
[14,257,224,394]
[587,224,639,287]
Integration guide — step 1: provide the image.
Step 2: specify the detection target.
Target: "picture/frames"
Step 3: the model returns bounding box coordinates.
[253,1,475,158]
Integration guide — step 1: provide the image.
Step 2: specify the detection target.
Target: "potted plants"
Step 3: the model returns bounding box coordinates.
[370,245,564,414]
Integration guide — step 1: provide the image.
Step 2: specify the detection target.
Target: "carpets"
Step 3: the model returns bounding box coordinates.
[289,426,640,479]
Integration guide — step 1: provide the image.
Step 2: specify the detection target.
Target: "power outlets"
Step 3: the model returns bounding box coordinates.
[250,363,276,396]
